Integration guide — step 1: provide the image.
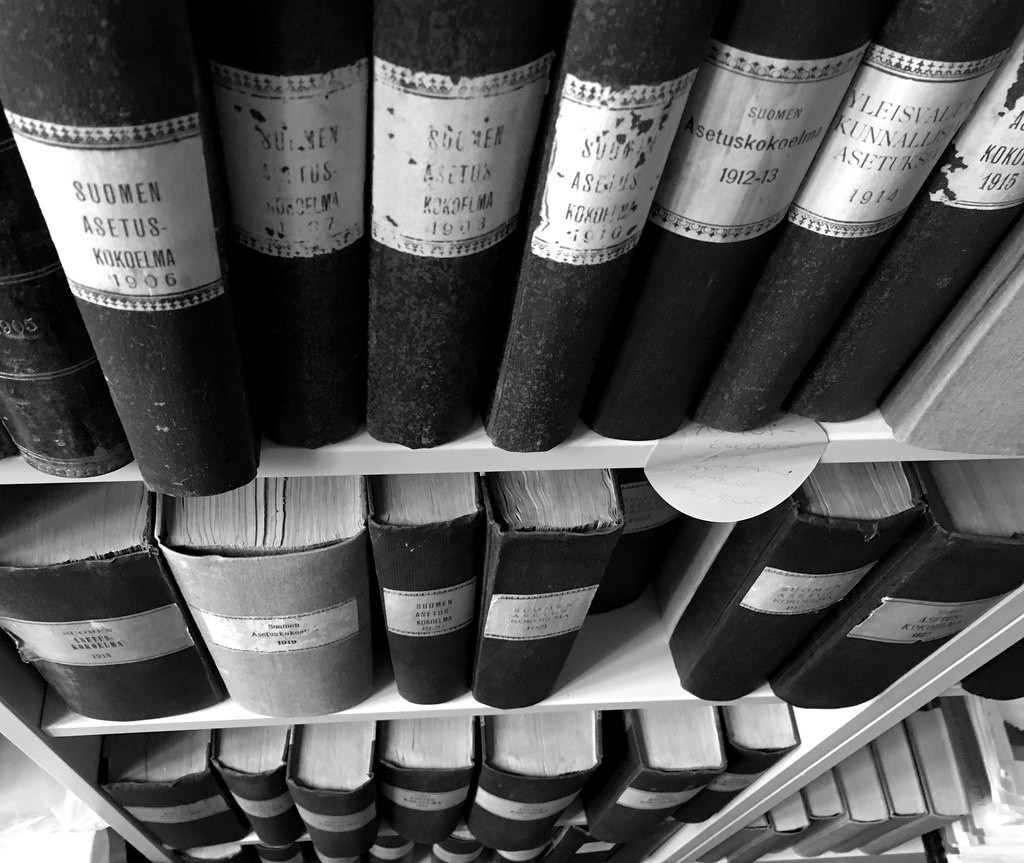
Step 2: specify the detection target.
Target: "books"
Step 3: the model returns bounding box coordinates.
[155,476,380,720]
[1,0,1024,863]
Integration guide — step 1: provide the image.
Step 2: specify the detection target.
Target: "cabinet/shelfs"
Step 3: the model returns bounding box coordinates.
[0,0,1024,863]
